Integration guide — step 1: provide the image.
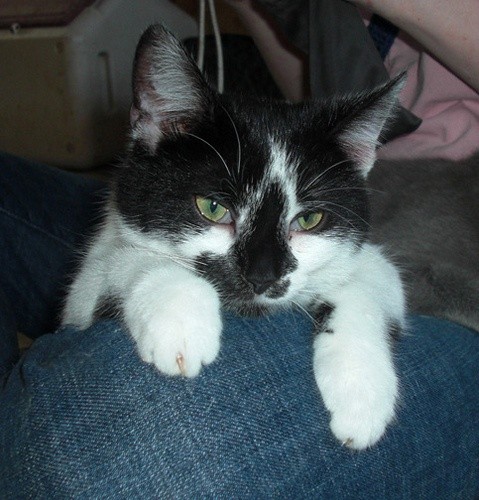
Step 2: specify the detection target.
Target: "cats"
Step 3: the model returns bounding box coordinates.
[56,24,479,450]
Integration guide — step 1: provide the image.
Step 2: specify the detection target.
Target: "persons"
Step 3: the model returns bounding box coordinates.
[0,0,479,500]
